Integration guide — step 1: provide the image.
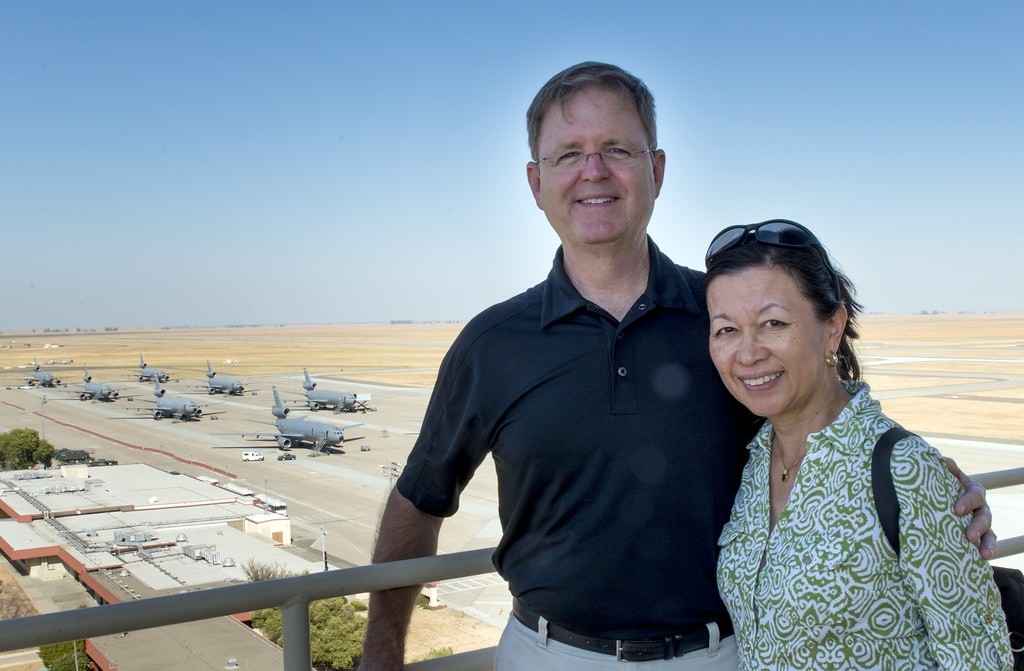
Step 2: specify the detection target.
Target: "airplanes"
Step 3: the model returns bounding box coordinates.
[59,363,136,401]
[126,353,170,383]
[209,386,365,457]
[16,358,61,388]
[123,375,225,423]
[281,368,358,414]
[186,359,254,396]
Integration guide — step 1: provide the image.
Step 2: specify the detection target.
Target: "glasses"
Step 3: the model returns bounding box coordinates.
[705,219,841,301]
[534,140,656,173]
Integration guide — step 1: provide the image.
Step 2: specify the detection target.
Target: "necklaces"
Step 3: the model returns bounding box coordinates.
[775,433,808,483]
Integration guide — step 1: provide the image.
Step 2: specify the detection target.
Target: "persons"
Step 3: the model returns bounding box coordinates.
[357,61,996,671]
[705,219,1015,671]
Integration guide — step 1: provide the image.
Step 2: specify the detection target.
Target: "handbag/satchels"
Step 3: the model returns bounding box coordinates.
[868,427,1024,671]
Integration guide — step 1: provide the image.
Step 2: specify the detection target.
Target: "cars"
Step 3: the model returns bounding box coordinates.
[277,453,296,461]
[243,452,264,461]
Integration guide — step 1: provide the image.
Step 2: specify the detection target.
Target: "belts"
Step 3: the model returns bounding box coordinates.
[512,597,735,662]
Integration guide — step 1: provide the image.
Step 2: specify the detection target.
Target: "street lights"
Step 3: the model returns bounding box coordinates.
[40,394,46,439]
[320,526,327,570]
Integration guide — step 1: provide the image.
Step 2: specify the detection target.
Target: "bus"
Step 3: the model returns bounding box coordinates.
[222,483,254,496]
[254,494,288,517]
